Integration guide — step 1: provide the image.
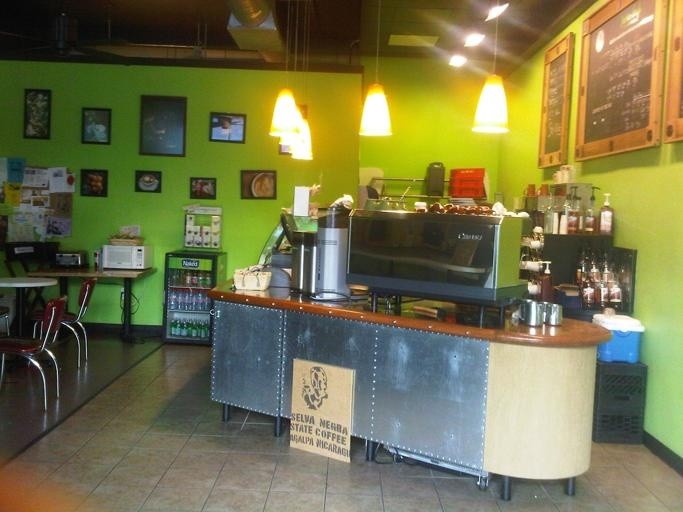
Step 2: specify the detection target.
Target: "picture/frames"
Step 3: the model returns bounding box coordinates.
[23,87,308,199]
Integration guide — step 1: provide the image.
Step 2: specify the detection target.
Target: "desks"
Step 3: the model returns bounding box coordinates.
[28,267,154,347]
[0,276,58,373]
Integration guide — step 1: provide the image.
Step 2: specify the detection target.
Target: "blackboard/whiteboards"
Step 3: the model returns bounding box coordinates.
[574,0,683,163]
[539,32,574,169]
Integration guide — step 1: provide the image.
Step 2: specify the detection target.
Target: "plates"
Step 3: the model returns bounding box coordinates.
[249,174,273,197]
[88,127,109,139]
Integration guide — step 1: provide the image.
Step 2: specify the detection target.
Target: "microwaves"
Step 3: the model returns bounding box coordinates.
[101,244,153,270]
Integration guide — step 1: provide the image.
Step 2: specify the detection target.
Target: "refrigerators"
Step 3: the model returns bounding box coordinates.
[161,248,229,346]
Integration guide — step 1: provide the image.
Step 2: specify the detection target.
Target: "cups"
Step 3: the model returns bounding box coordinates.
[317,207,352,302]
[519,298,563,327]
[138,176,160,192]
[142,174,156,186]
[524,182,548,196]
[94,124,106,136]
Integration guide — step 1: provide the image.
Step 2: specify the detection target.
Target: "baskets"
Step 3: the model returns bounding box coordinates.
[107,236,144,246]
[233,270,272,292]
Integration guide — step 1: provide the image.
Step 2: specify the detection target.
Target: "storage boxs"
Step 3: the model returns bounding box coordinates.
[592,314,645,364]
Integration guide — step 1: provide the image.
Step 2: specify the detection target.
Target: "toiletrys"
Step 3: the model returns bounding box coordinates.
[583,185,601,232]
[540,261,552,301]
[564,184,582,234]
[599,193,614,232]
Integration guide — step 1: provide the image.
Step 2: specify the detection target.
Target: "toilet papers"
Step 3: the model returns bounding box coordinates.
[519,259,543,272]
[294,186,310,216]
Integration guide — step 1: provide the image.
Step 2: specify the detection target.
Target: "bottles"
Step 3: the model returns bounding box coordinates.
[576,248,624,312]
[169,316,209,339]
[168,289,212,311]
[290,228,318,299]
[544,206,553,234]
[94,248,103,272]
[568,208,596,235]
[168,268,212,288]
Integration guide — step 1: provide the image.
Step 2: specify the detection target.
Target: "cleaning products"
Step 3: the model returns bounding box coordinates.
[576,256,622,310]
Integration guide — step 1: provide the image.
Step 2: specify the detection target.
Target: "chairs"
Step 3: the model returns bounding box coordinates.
[29,279,97,369]
[0,307,10,337]
[0,295,67,411]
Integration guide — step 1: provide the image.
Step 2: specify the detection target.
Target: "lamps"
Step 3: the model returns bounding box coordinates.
[471,0,510,134]
[359,0,393,138]
[269,0,314,161]
